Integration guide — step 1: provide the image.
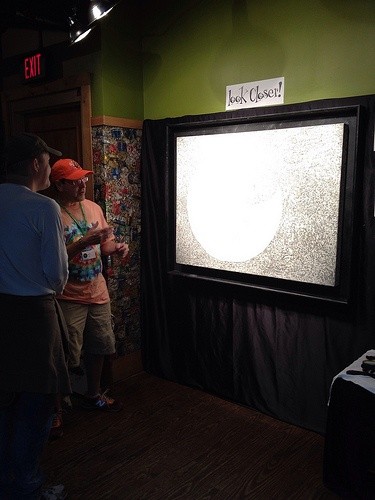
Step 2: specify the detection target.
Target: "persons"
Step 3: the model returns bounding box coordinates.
[49,158,130,421]
[0,132,70,500]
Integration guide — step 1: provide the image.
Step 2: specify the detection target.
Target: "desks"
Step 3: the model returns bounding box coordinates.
[322,349,375,500]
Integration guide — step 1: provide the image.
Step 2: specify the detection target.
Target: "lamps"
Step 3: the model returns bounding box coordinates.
[87,0,120,28]
[63,6,96,48]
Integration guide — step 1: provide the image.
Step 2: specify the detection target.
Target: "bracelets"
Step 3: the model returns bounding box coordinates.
[79,239,86,250]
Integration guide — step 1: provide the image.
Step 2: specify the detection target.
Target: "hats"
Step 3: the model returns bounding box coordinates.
[6,131,63,165]
[51,159,94,181]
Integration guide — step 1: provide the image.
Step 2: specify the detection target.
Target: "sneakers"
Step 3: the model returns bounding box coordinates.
[83,393,125,412]
[47,415,63,440]
[26,484,67,500]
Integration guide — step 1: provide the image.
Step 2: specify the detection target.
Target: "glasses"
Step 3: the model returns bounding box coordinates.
[60,177,90,186]
